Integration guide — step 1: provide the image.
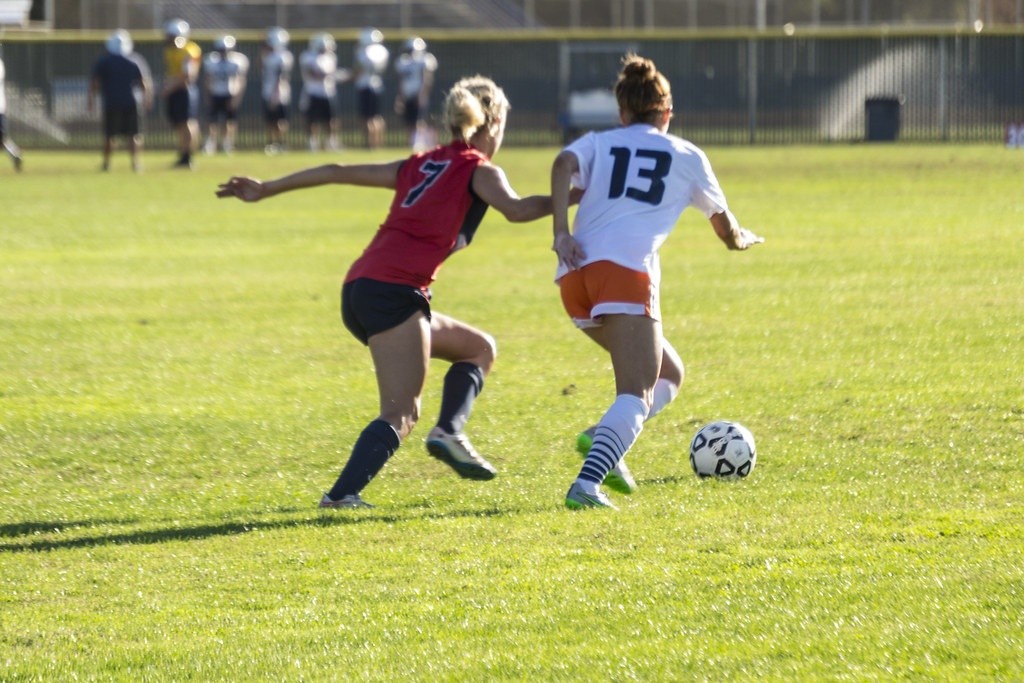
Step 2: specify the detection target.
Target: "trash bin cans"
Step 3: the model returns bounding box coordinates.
[865,96,901,141]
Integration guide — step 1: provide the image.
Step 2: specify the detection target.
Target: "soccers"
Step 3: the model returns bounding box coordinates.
[688,420,758,484]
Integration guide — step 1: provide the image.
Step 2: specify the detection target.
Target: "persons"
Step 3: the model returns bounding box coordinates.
[299,32,350,150]
[552,58,763,513]
[216,75,583,510]
[161,19,201,167]
[0,60,23,170]
[203,35,249,156]
[86,27,153,171]
[260,26,294,154]
[395,37,437,146]
[353,28,392,149]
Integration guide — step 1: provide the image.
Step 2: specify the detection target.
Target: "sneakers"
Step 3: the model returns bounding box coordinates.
[565,482,618,512]
[577,425,638,494]
[318,493,371,508]
[425,426,496,481]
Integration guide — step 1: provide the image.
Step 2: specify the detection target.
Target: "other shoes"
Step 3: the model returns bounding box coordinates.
[173,156,191,169]
[13,156,22,170]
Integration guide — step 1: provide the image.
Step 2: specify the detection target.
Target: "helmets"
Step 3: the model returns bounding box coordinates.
[267,27,289,47]
[312,33,334,48]
[166,19,190,38]
[406,37,426,51]
[362,29,383,44]
[216,35,236,49]
[106,29,132,55]
